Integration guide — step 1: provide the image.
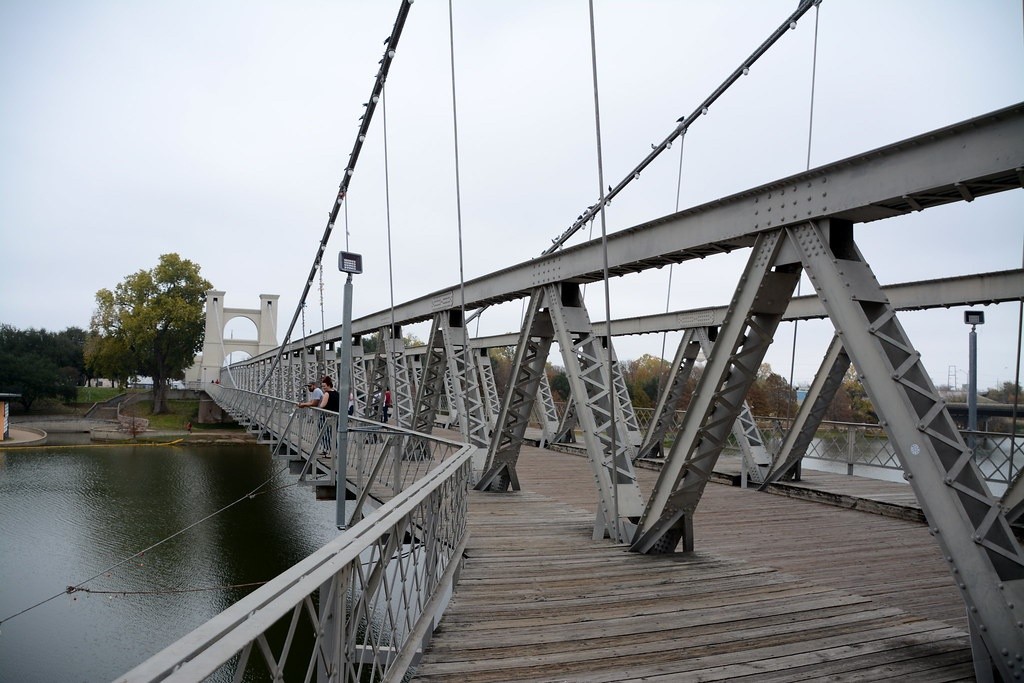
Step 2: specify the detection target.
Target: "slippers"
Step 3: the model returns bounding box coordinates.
[320,451,332,459]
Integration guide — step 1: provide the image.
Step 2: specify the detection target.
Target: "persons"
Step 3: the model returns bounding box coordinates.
[319,376,341,459]
[372,387,391,423]
[186,422,192,434]
[347,392,354,422]
[298,382,327,455]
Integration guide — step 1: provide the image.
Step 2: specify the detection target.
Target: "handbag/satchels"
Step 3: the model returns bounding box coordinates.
[387,408,394,415]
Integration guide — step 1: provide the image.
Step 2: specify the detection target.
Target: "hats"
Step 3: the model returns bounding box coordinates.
[304,381,317,386]
